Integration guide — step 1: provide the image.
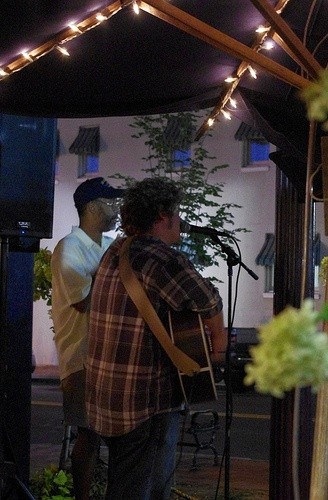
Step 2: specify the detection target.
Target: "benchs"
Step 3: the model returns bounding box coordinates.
[56,408,222,469]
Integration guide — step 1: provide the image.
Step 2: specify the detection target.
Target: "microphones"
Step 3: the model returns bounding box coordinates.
[180,220,230,238]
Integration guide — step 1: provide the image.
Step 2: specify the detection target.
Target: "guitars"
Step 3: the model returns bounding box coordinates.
[168,298,218,414]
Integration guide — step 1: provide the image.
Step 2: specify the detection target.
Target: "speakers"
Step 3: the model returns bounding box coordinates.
[0,117,57,238]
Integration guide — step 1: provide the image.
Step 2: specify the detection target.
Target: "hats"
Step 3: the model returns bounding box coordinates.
[73,177,127,207]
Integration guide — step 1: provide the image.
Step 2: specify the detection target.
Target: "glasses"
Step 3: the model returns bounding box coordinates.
[95,198,118,207]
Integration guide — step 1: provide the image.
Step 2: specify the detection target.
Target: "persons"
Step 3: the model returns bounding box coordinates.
[50,176,121,500]
[84,177,225,500]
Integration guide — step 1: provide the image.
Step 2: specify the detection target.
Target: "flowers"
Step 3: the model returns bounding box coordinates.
[241,297,328,398]
